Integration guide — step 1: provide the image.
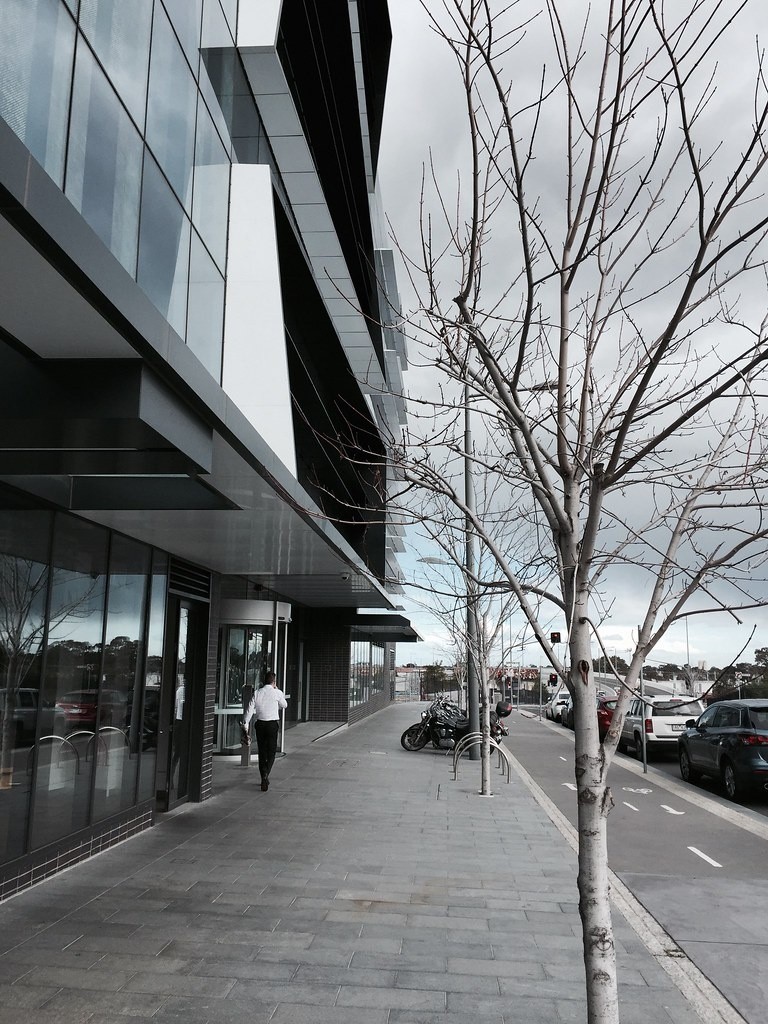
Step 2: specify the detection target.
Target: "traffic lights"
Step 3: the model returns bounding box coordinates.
[551,632,560,643]
[550,674,558,687]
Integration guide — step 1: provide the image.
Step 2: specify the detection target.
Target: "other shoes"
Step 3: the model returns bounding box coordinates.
[171,780,174,789]
[261,775,270,792]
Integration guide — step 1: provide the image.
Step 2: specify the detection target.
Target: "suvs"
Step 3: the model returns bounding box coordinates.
[1,687,70,748]
[618,694,708,765]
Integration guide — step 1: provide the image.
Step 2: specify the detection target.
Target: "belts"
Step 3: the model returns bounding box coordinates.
[256,720,277,724]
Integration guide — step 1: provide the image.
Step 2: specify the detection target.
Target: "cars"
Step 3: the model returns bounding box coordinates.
[596,696,621,744]
[678,698,768,801]
[544,690,570,723]
[560,692,613,731]
[55,687,130,734]
[350,671,384,702]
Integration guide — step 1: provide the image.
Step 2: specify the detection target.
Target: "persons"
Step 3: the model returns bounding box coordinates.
[169,671,186,789]
[242,671,288,792]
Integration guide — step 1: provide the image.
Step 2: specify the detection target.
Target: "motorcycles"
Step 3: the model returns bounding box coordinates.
[117,689,161,752]
[400,694,513,758]
